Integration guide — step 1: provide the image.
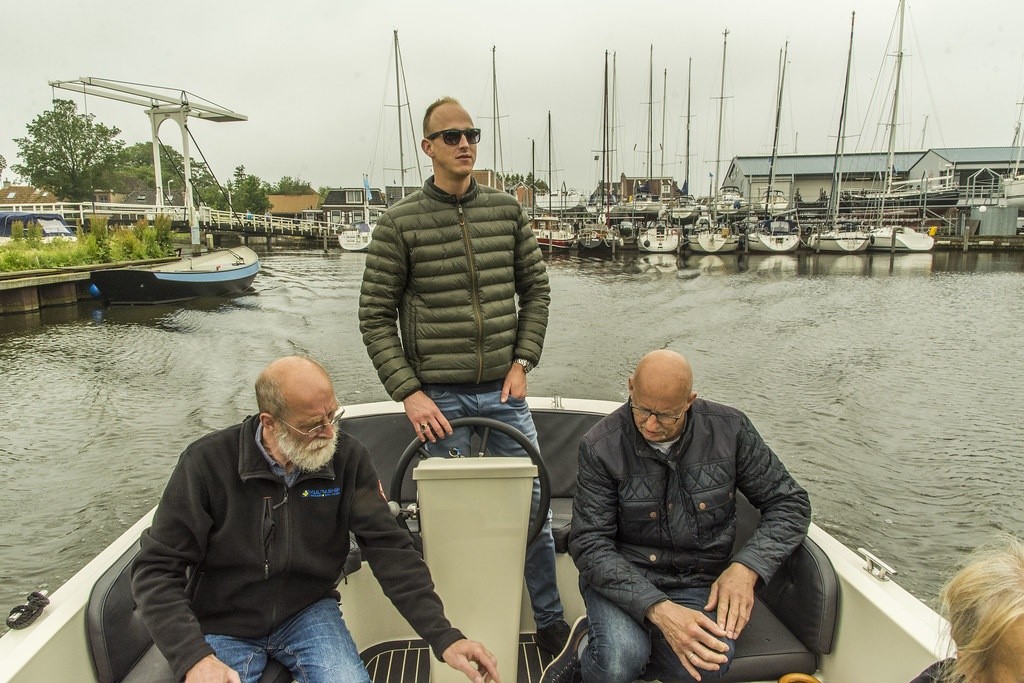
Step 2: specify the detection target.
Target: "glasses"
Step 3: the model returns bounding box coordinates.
[274,395,346,437]
[628,393,687,425]
[427,127,481,145]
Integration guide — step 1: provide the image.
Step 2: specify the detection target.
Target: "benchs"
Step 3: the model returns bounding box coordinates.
[335,409,610,557]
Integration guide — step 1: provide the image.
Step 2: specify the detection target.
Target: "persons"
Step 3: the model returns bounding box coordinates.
[132,355,502,683]
[537,348,812,683]
[905,534,1024,683]
[359,97,571,658]
[293,212,301,231]
[264,209,272,229]
[246,209,252,226]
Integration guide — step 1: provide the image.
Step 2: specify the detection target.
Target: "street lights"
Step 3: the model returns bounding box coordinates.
[167,180,174,206]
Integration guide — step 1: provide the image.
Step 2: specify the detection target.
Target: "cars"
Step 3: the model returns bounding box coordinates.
[136,194,146,200]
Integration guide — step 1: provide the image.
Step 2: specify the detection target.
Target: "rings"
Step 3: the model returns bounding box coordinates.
[688,653,695,660]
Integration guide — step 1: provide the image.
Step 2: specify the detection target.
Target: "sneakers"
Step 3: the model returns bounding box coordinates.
[534,619,571,657]
[540,614,588,683]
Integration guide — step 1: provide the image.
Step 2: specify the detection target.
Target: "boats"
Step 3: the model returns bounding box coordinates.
[89,244,262,308]
[0,211,80,249]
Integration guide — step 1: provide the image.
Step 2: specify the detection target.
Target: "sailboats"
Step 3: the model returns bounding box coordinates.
[337,30,425,253]
[1001,95,1024,200]
[478,0,936,254]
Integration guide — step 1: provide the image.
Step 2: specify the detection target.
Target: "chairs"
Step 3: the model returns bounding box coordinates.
[725,489,841,683]
[84,538,293,683]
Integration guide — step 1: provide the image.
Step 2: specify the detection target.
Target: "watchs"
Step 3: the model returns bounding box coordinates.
[512,357,533,373]
